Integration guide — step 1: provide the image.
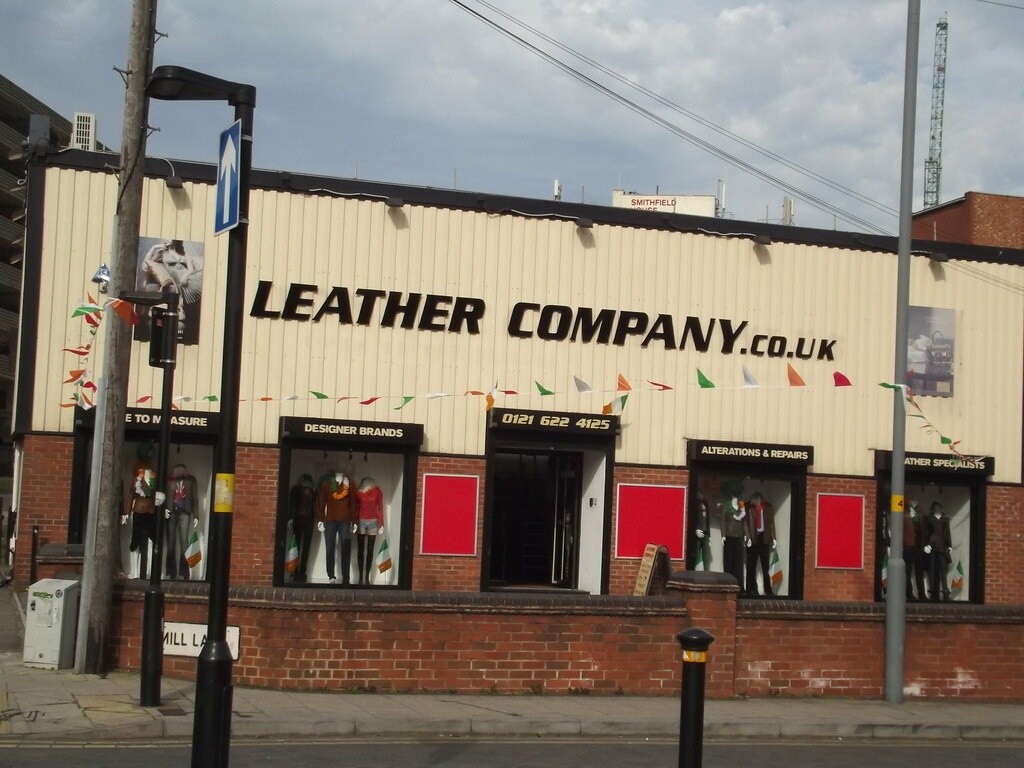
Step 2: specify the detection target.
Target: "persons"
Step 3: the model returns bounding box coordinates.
[288,471,386,587]
[882,498,952,600]
[123,461,200,579]
[695,489,780,597]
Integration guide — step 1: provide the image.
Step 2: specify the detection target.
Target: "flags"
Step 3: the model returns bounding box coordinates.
[286,535,299,575]
[881,555,888,592]
[768,547,783,584]
[375,540,392,574]
[184,532,202,567]
[951,561,965,589]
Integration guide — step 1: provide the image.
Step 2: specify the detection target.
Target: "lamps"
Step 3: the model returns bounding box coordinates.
[166,176,183,189]
[750,235,770,244]
[574,219,593,228]
[927,253,948,262]
[386,197,403,208]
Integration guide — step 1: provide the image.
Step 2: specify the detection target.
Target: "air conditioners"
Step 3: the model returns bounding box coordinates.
[72,112,96,153]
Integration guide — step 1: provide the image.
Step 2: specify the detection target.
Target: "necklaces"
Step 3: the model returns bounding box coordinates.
[330,474,349,499]
[135,466,156,496]
[727,498,746,519]
